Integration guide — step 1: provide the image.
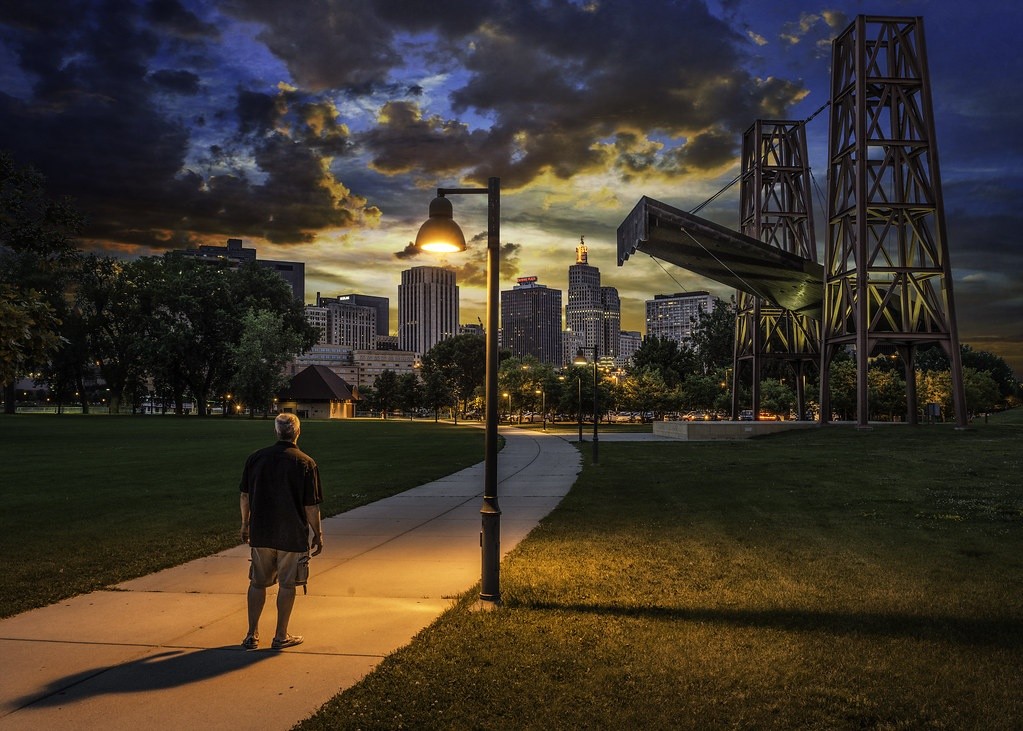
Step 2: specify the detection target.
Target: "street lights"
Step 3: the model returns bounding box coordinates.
[414,177,508,611]
[503,393,512,426]
[557,372,583,442]
[535,390,546,432]
[572,344,600,468]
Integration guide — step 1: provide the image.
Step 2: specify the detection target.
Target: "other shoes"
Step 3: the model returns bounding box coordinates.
[271,633,304,647]
[243,633,259,650]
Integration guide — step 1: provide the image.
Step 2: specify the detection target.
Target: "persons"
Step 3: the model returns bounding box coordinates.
[239,414,323,649]
